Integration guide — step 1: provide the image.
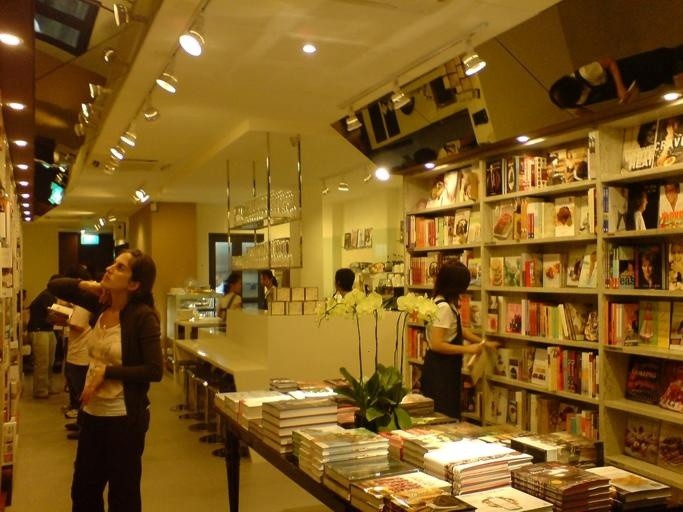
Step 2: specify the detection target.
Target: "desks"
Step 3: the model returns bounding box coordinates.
[202,380,683,512]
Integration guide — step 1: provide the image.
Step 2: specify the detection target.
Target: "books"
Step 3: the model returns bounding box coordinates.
[408,114,682,352]
[215,358,683,512]
[619,80,640,105]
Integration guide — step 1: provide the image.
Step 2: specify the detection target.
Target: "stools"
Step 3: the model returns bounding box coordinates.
[167,354,250,463]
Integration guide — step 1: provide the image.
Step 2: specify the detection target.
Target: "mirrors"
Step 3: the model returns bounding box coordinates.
[326,0,683,175]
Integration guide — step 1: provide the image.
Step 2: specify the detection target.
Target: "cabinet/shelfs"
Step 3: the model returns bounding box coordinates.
[390,88,683,510]
[0,108,25,510]
[160,289,225,385]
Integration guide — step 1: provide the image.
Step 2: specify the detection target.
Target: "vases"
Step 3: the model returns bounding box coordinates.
[333,398,367,430]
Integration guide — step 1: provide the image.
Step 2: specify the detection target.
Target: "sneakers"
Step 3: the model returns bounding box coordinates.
[62,403,79,439]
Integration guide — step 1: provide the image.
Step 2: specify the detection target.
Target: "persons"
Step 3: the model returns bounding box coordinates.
[18,266,105,440]
[334,268,355,298]
[549,45,683,109]
[218,271,242,333]
[422,259,472,424]
[48,251,164,512]
[261,269,278,310]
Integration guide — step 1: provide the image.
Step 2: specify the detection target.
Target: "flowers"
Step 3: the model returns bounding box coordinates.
[306,274,453,441]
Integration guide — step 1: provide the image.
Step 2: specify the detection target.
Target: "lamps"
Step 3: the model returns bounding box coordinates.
[307,156,382,201]
[102,158,119,176]
[156,71,181,94]
[120,130,138,147]
[180,28,209,57]
[143,107,160,120]
[331,20,495,136]
[81,102,102,117]
[102,48,121,67]
[87,187,151,231]
[110,147,125,160]
[89,83,114,98]
[112,3,148,28]
[73,123,93,137]
